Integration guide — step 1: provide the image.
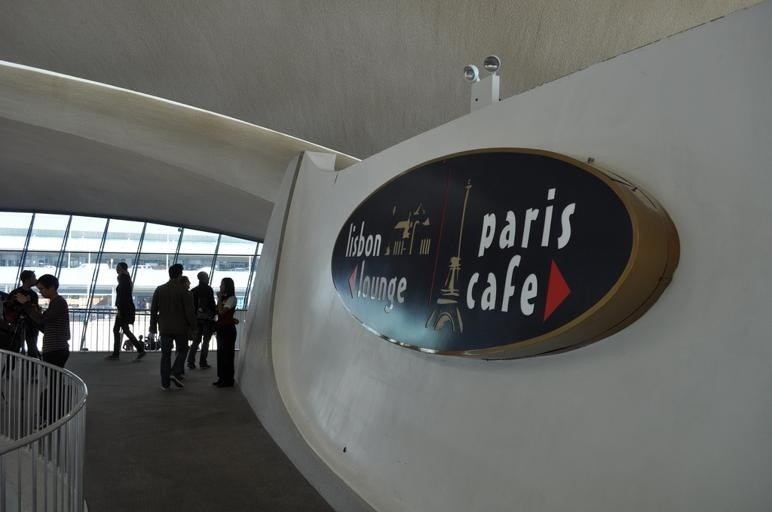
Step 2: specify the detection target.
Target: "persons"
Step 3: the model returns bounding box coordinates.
[102,262,146,360]
[123,262,240,388]
[10,270,40,386]
[17,275,70,432]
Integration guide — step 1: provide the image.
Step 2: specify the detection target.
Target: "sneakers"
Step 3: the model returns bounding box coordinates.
[104,354,120,360]
[135,351,147,360]
[159,381,171,392]
[169,374,184,389]
[186,361,197,370]
[212,378,235,387]
[33,421,50,432]
[199,361,212,368]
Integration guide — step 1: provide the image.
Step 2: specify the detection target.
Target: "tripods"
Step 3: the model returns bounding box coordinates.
[0,316,44,378]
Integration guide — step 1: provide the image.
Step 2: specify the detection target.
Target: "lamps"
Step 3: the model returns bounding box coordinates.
[461,53,502,113]
